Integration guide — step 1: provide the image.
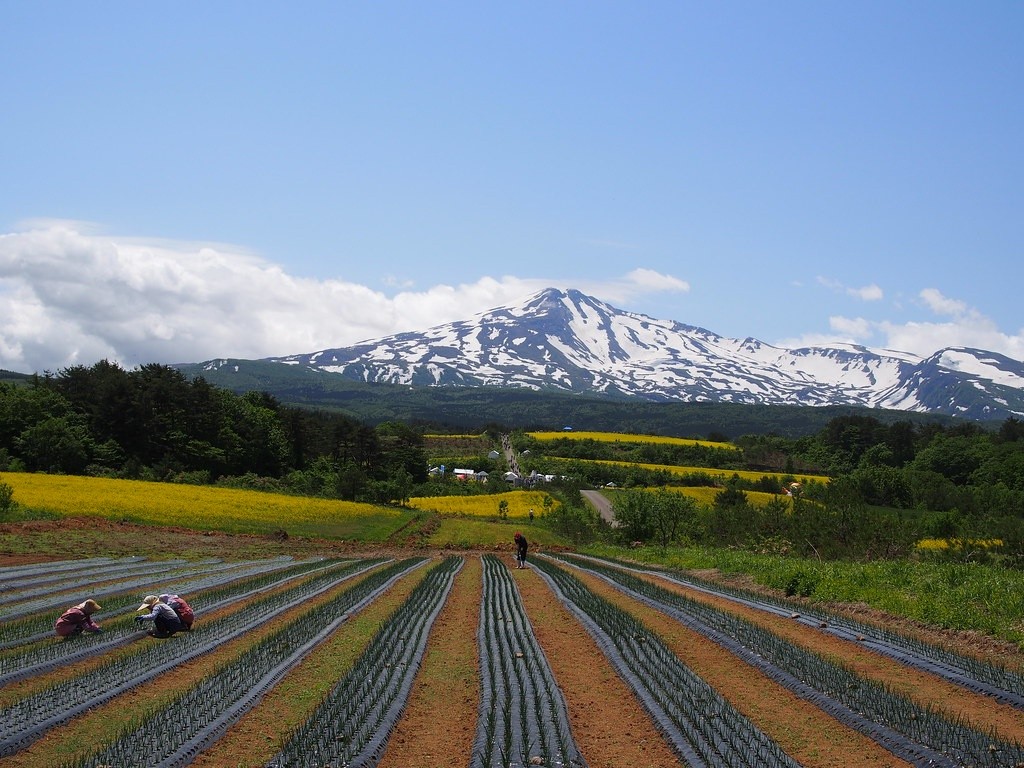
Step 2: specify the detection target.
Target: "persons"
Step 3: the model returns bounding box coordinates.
[134,593,194,636]
[514,530,528,569]
[530,509,533,516]
[55,599,103,637]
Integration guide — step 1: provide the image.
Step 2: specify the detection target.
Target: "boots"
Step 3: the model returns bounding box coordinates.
[519,560,525,569]
[515,560,521,569]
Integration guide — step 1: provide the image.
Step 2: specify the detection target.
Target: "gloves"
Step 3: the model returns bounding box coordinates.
[133,615,145,626]
[95,628,103,634]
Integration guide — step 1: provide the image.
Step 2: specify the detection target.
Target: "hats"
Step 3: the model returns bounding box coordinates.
[159,593,168,603]
[515,533,521,538]
[136,595,160,611]
[74,600,101,613]
